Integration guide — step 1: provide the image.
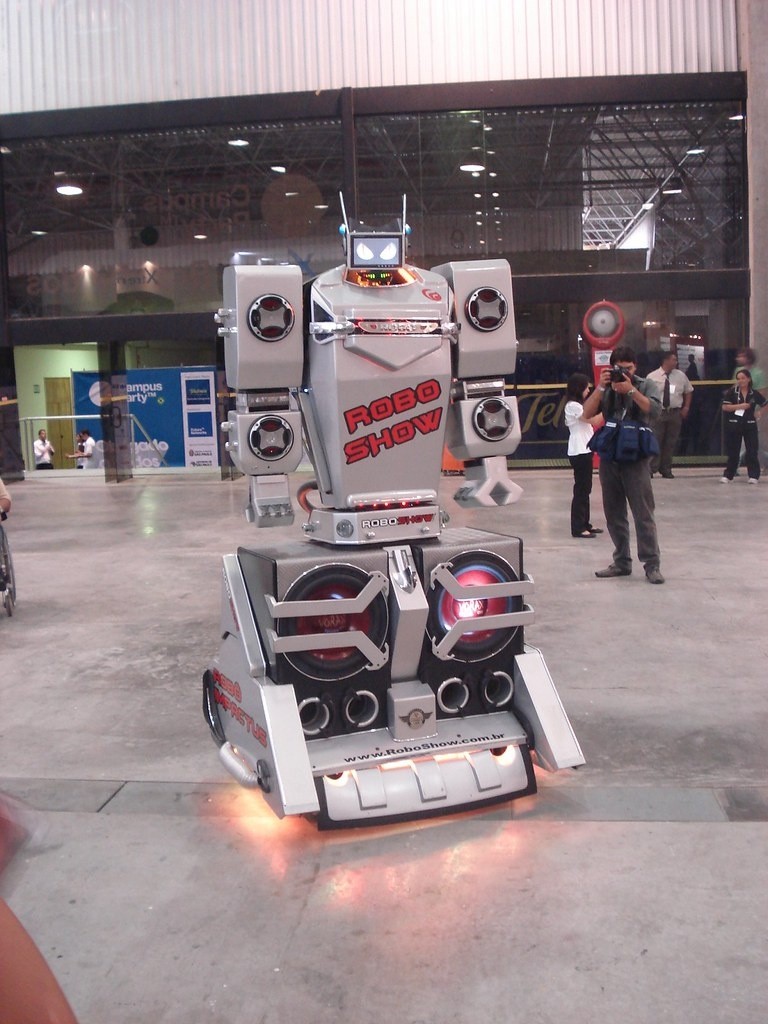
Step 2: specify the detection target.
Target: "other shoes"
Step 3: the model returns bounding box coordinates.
[587,526,602,533]
[646,568,663,584]
[572,530,596,538]
[659,470,675,479]
[595,566,632,578]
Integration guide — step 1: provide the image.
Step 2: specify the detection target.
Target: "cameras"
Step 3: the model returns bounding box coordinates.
[608,365,631,382]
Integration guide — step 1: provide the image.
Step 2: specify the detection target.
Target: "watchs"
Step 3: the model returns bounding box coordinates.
[627,387,636,396]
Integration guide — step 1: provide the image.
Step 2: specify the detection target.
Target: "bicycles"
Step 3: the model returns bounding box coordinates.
[0,507,17,617]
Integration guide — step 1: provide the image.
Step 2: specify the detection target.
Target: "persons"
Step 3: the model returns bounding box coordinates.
[65,430,95,469]
[685,354,697,380]
[201,190,587,823]
[582,347,665,584]
[719,348,768,484]
[645,351,694,478]
[0,478,16,609]
[564,374,605,539]
[33,429,55,469]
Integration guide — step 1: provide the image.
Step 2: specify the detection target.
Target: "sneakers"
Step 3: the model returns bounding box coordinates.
[719,477,735,483]
[748,477,758,484]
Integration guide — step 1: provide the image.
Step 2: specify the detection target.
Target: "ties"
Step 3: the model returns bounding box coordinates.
[663,375,670,410]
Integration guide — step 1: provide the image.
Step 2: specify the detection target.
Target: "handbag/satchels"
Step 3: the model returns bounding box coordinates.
[586,420,660,462]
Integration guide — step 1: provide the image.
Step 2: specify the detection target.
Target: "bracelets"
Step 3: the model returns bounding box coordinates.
[597,385,605,391]
